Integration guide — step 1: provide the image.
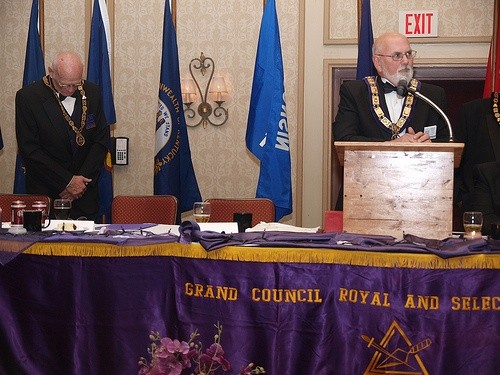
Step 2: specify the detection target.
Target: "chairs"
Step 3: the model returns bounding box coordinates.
[204,198,276,226]
[111,194,177,224]
[0,193,52,222]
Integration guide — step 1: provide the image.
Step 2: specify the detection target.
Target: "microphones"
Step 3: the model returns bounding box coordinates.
[397,77,407,95]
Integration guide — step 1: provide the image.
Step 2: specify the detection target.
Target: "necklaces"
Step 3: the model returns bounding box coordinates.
[362,76,422,141]
[41,74,87,147]
[491,92,500,126]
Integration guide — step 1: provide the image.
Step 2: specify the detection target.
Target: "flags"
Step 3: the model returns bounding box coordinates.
[12,0,47,193]
[481,0,500,100]
[87,0,116,221]
[153,0,202,224]
[245,1,293,224]
[356,0,375,83]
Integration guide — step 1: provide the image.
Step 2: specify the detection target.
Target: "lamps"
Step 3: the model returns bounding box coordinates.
[182,52,232,128]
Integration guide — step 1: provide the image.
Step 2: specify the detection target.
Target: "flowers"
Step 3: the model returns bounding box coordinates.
[138,320,266,375]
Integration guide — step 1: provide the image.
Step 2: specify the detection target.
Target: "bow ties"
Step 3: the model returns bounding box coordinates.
[381,82,408,97]
[59,91,78,101]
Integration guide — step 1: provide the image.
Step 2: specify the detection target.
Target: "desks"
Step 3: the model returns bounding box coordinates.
[0,224,500,375]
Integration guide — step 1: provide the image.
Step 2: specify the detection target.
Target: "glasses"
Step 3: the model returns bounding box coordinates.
[53,72,82,87]
[376,49,417,61]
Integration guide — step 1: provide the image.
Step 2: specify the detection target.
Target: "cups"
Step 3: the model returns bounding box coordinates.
[193,202,212,222]
[22,210,51,232]
[54,199,71,220]
[463,211,484,233]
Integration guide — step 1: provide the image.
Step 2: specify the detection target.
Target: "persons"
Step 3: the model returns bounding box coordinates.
[331,32,450,212]
[452,92,500,241]
[15,51,110,223]
